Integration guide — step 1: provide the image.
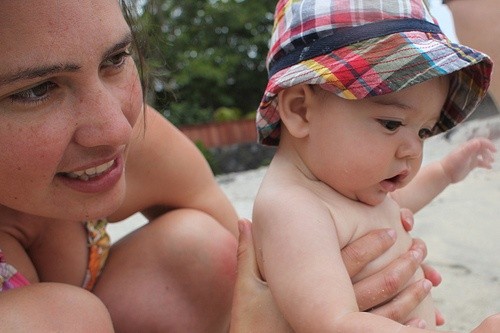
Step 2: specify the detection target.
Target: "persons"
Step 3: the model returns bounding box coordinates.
[249,0,500,332]
[0,0,500,333]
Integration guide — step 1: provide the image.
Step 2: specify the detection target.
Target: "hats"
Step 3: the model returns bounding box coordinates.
[255,0,494,148]
